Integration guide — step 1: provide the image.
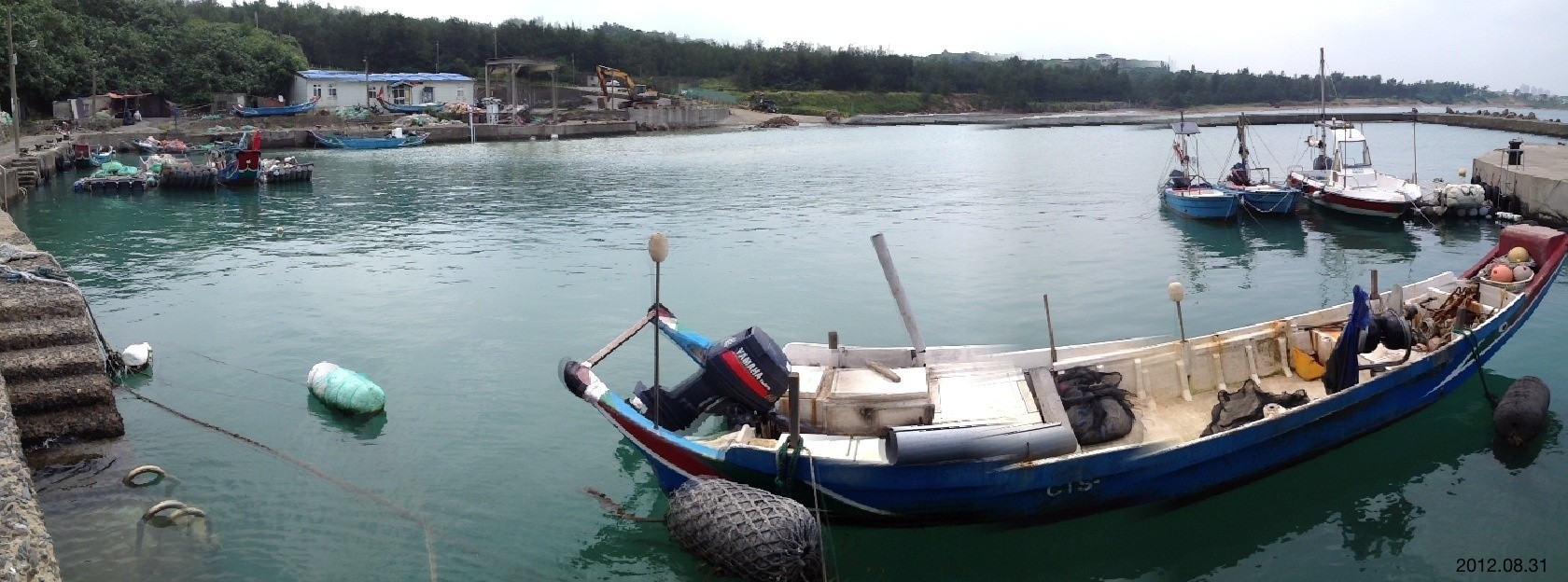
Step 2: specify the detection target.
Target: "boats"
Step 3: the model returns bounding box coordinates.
[1157,104,1240,220]
[1284,43,1423,223]
[556,221,1567,529]
[1218,110,1300,215]
[66,144,115,167]
[376,97,446,113]
[232,96,319,116]
[131,139,214,154]
[305,130,432,148]
[206,142,260,184]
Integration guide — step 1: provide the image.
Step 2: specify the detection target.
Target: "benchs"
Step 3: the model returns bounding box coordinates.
[1029,366,1081,454]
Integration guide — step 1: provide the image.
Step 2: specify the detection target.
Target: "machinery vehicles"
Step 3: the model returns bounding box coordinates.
[739,92,778,113]
[596,65,662,109]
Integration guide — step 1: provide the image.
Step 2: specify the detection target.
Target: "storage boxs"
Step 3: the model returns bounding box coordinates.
[774,364,929,436]
